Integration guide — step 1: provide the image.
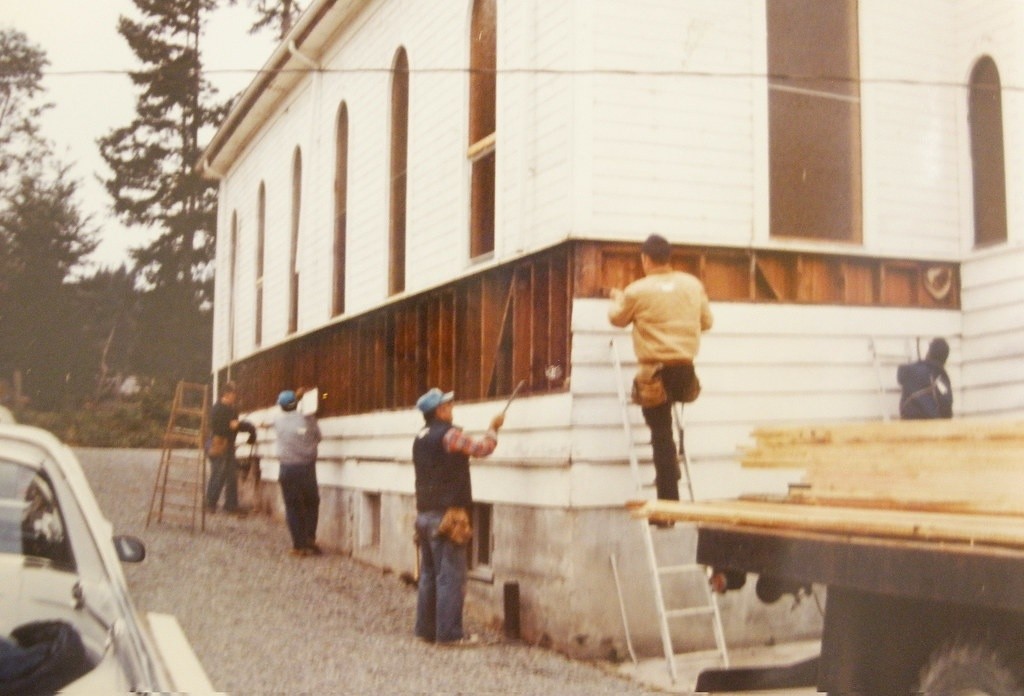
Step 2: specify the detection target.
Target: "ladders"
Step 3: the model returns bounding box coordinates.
[145,381,212,539]
[607,336,731,688]
[872,341,920,420]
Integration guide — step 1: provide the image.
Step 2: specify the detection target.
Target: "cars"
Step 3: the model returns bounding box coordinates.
[0,420,221,696]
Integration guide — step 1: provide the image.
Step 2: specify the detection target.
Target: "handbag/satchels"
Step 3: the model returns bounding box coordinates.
[634,362,667,409]
[441,509,470,546]
[208,436,227,457]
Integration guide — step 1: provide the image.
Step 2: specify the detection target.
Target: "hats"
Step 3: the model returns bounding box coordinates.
[416,387,454,415]
[930,339,948,358]
[278,390,296,407]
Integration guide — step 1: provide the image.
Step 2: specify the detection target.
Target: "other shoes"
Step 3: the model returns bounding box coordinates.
[288,543,319,557]
[206,503,217,513]
[223,502,240,513]
[647,517,675,529]
[436,632,477,648]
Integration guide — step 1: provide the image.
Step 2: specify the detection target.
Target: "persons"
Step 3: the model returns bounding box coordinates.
[412,387,505,647]
[276,387,322,557]
[609,234,714,529]
[896,336,953,421]
[203,383,256,517]
